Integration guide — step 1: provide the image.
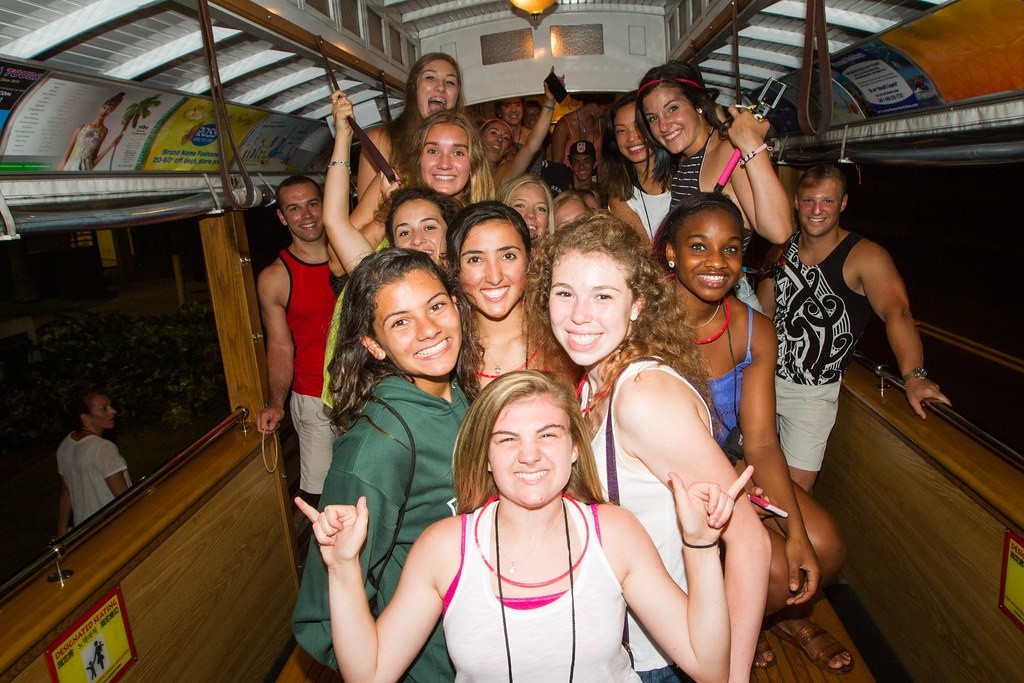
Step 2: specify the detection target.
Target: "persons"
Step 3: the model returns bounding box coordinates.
[257,47,597,683]
[521,62,952,683]
[54,383,134,542]
[56,92,126,172]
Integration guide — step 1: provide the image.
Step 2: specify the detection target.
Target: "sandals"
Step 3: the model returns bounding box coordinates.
[752,629,776,669]
[769,614,855,675]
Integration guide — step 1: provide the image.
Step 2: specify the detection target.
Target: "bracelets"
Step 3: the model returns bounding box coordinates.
[740,143,767,165]
[544,104,555,111]
[327,160,351,174]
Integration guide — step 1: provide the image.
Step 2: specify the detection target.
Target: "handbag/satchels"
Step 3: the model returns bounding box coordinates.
[540,160,573,198]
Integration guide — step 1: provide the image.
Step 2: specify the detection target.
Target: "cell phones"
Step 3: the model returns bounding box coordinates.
[545,73,568,105]
[747,494,788,518]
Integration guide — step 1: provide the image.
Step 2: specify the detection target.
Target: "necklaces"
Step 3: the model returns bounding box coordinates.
[483,327,516,374]
[474,490,588,588]
[471,349,539,378]
[574,373,612,415]
[663,272,732,345]
[578,116,589,133]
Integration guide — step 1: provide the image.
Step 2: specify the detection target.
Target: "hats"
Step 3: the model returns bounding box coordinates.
[567,141,596,163]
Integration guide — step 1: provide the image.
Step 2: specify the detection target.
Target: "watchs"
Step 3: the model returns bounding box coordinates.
[903,368,927,386]
